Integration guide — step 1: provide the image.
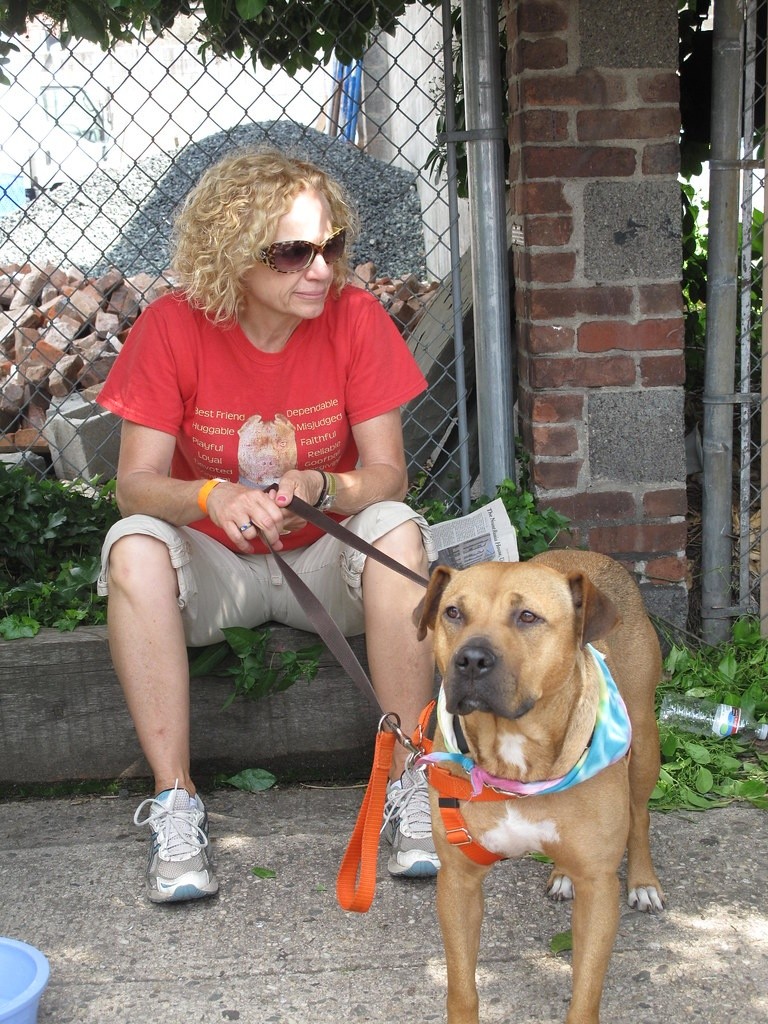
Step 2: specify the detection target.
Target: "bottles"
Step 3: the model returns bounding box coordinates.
[658,693,768,740]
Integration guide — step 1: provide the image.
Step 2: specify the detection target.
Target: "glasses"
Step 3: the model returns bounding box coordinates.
[261,221,346,274]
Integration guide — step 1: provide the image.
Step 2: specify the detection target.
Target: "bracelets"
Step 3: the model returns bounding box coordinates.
[313,471,326,507]
[198,478,228,515]
[240,521,253,533]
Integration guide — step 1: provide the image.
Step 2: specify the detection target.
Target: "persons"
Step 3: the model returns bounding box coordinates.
[98,145,441,903]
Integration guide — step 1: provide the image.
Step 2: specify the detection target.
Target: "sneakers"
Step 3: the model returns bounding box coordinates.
[134,778,219,902]
[378,767,442,878]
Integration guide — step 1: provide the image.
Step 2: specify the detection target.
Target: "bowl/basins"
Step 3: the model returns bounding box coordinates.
[0,937,51,1023]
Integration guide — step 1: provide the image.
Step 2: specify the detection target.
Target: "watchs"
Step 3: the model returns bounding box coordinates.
[319,472,336,513]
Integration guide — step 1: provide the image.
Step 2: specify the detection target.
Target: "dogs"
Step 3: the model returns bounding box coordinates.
[412,549,667,1024]
